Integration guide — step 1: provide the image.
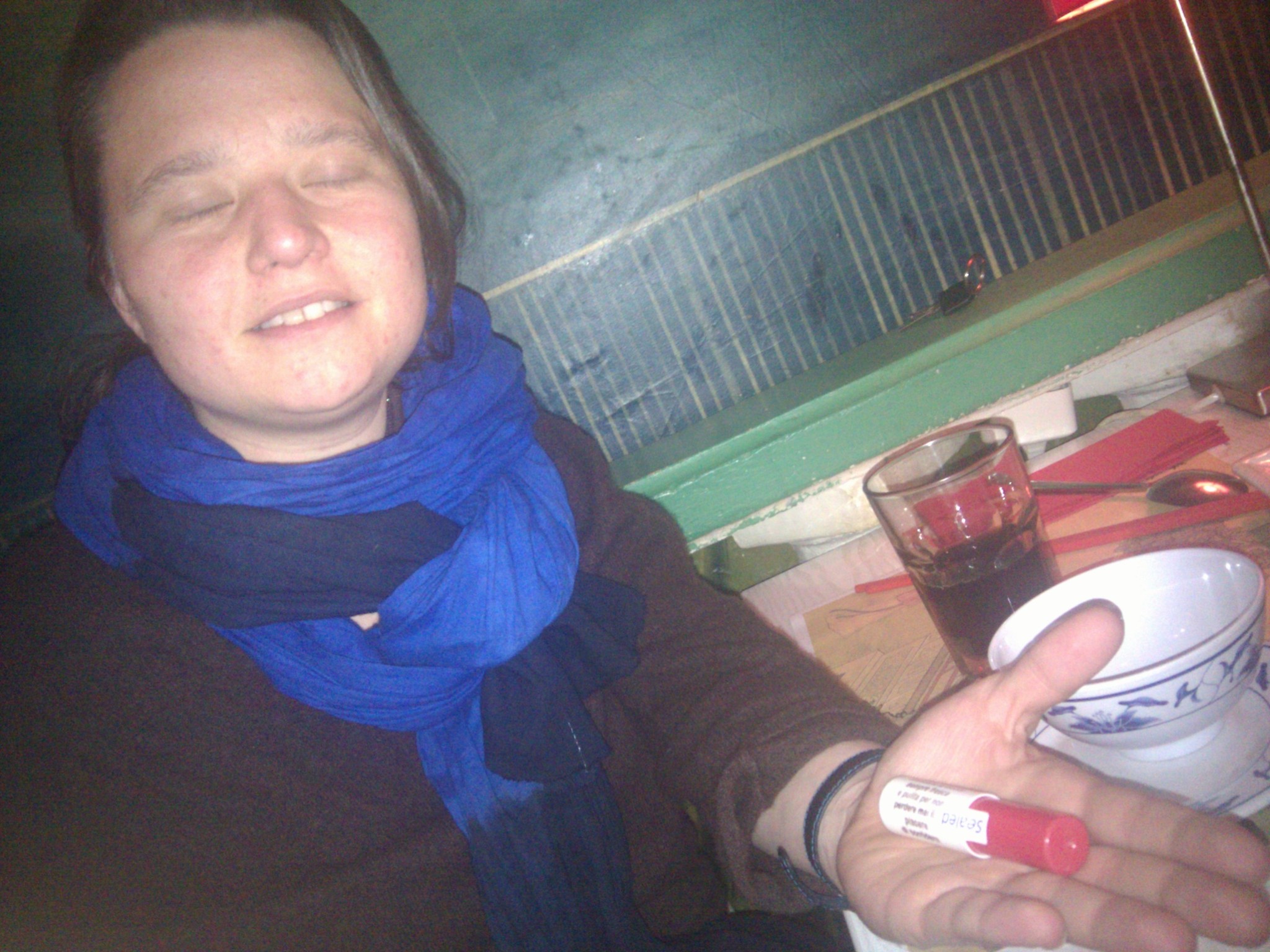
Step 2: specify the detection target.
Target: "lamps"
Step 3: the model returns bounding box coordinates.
[1043,0,1269,272]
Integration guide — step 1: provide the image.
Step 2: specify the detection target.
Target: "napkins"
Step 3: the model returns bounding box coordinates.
[1030,407,1229,522]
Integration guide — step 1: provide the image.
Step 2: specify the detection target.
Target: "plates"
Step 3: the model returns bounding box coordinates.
[1029,640,1269,822]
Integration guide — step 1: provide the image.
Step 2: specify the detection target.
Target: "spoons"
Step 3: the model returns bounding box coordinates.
[1030,467,1248,509]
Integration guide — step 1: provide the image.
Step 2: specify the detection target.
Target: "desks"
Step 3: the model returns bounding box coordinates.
[740,384,1270,952]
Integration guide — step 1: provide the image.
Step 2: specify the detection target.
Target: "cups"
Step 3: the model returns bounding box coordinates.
[863,417,1063,683]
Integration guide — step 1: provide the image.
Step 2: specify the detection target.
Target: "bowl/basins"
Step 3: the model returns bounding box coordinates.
[987,547,1266,764]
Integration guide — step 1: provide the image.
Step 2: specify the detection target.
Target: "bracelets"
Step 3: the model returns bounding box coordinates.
[776,748,885,912]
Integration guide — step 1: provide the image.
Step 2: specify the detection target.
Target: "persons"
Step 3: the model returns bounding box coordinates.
[0,0,1270,952]
